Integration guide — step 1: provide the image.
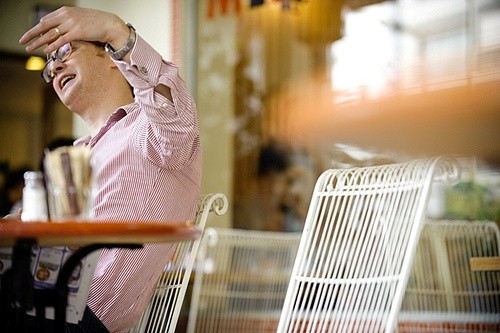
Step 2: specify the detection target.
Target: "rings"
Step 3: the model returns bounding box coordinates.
[55,27,62,36]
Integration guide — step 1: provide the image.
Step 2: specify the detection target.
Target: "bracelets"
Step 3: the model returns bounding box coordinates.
[105,22,137,59]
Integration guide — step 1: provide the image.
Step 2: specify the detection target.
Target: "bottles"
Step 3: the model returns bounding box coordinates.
[21,171,49,223]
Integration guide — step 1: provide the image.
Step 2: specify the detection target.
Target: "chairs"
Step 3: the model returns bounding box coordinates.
[185,223,307,333]
[391,218,499,333]
[277,151,463,332]
[126,191,228,332]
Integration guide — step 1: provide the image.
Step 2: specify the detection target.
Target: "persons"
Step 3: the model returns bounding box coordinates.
[0,6,202,333]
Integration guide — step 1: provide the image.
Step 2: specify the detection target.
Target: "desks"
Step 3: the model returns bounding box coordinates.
[0,218,203,332]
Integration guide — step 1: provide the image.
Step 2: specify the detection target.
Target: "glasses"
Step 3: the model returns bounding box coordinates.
[41,41,73,84]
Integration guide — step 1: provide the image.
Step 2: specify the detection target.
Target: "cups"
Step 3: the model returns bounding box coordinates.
[43,146,97,221]
